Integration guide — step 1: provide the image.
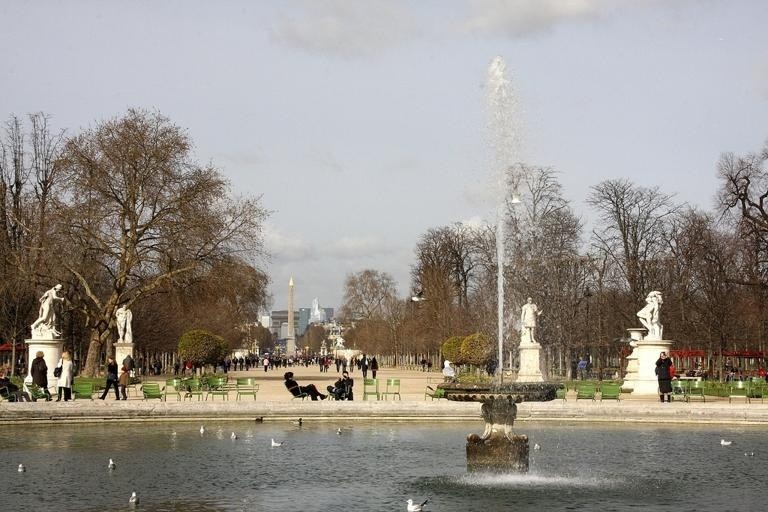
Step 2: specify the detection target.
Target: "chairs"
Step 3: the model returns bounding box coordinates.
[283,379,402,401]
[555,378,621,400]
[0,373,261,403]
[671,377,768,402]
[425,385,446,400]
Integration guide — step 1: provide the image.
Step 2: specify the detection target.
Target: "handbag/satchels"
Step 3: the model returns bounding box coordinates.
[53,358,63,377]
[669,364,674,377]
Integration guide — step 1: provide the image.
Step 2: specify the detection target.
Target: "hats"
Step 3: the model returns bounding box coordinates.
[35,351,44,358]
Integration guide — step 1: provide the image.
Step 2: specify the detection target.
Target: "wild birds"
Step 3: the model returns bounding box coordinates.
[108,458,116,469]
[411,292,428,302]
[744,451,754,456]
[442,359,456,380]
[256,417,263,422]
[129,491,139,503]
[289,418,304,424]
[534,443,541,450]
[199,425,205,433]
[271,438,283,446]
[231,431,236,439]
[720,438,732,446]
[336,427,342,433]
[406,498,428,512]
[18,463,26,472]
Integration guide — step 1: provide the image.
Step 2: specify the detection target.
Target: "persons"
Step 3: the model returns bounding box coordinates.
[284,370,328,400]
[113,303,129,343]
[0,368,32,403]
[521,296,543,343]
[327,369,354,400]
[421,358,427,372]
[117,367,130,400]
[30,283,65,337]
[28,350,54,402]
[669,356,768,383]
[654,352,673,402]
[636,290,664,337]
[427,359,432,372]
[55,350,74,402]
[100,355,121,400]
[125,309,134,343]
[122,352,380,379]
